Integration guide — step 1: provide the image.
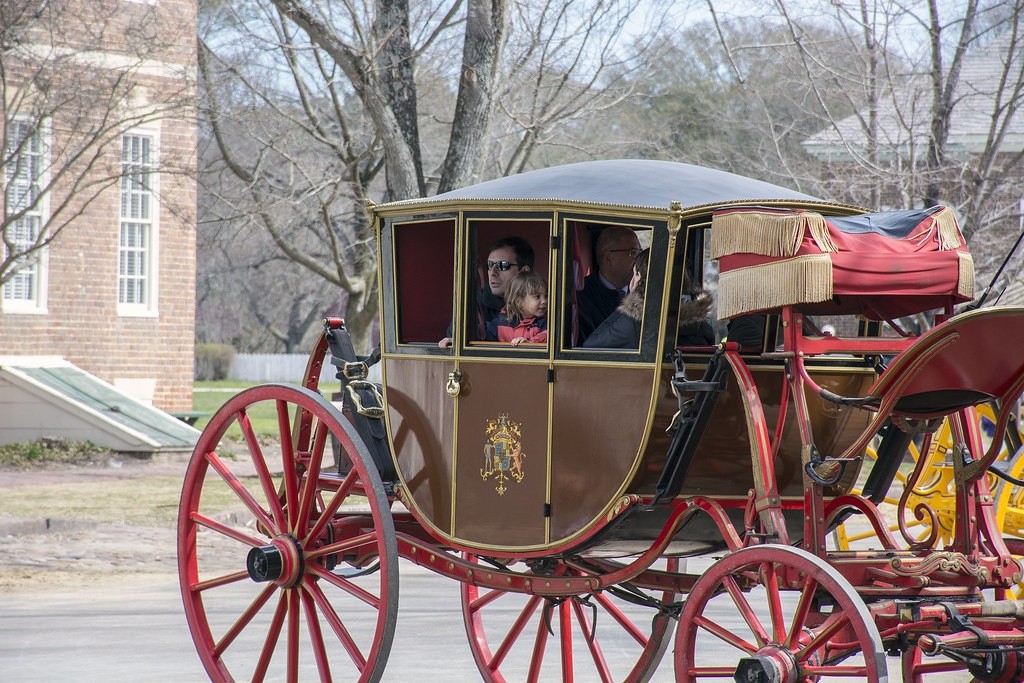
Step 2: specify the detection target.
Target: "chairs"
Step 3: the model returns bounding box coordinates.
[708,207,977,322]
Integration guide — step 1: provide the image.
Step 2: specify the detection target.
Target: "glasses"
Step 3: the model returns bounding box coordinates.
[611,249,643,257]
[486,261,521,271]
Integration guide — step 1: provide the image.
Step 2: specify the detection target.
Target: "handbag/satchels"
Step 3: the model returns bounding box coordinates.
[338,380,396,480]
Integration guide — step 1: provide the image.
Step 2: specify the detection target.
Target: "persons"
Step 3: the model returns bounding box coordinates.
[437,269,549,349]
[445,237,536,343]
[578,224,644,344]
[578,245,716,352]
